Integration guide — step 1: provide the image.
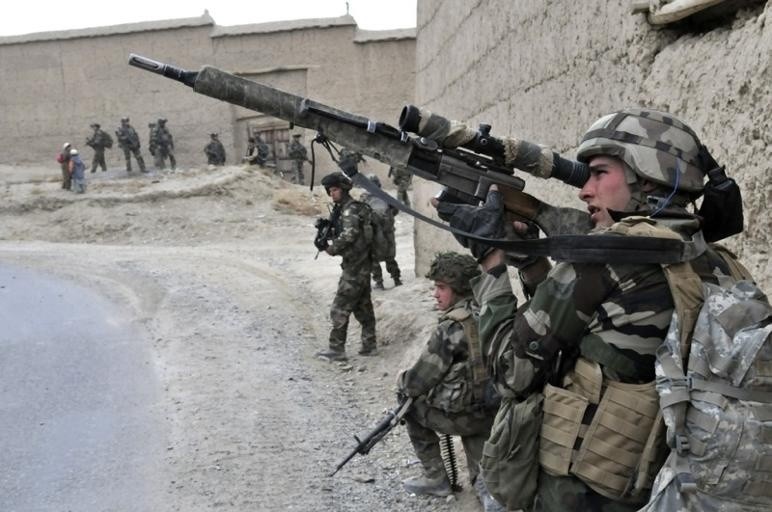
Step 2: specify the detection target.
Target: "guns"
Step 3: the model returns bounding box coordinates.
[328,392,416,476]
[86,137,96,149]
[314,203,341,261]
[287,141,313,165]
[127,53,595,260]
[116,127,136,146]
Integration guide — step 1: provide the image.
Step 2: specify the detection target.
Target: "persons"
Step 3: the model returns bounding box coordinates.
[357,172,406,289]
[395,248,511,512]
[428,104,759,511]
[54,112,314,193]
[384,161,417,209]
[313,171,384,361]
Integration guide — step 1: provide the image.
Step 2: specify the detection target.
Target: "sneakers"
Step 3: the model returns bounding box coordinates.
[360,348,378,356]
[320,350,344,360]
[403,475,453,496]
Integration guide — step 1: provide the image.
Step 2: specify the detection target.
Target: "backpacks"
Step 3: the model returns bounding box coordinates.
[640,245,772,512]
[345,199,393,256]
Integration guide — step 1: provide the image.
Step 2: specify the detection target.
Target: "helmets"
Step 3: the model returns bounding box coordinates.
[321,172,353,196]
[427,251,482,291]
[577,109,705,200]
